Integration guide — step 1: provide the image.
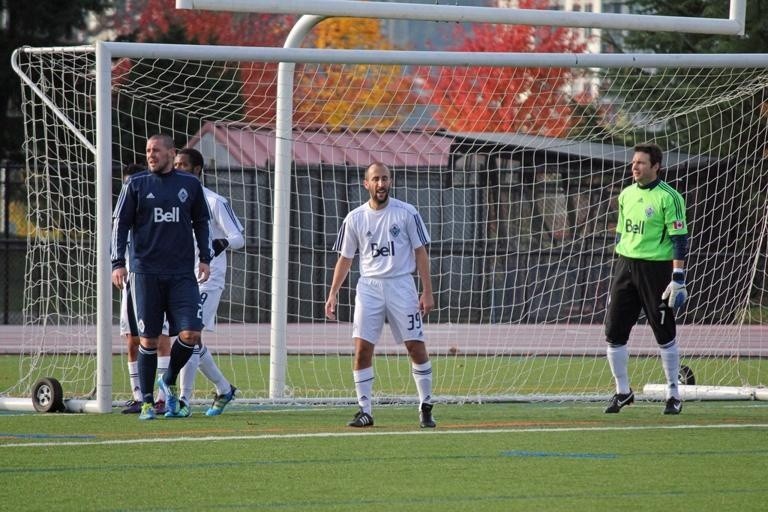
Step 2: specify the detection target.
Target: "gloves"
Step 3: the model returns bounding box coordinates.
[662,271,688,309]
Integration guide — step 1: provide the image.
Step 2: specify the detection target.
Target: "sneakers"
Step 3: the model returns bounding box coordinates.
[604,388,635,414]
[122,373,192,421]
[347,406,374,426]
[205,384,237,416]
[664,396,682,414]
[419,402,437,428]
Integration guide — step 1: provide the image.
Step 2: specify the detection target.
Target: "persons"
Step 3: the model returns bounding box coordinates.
[324,161,437,429]
[109,133,215,420]
[602,144,688,415]
[119,164,169,416]
[165,147,245,419]
